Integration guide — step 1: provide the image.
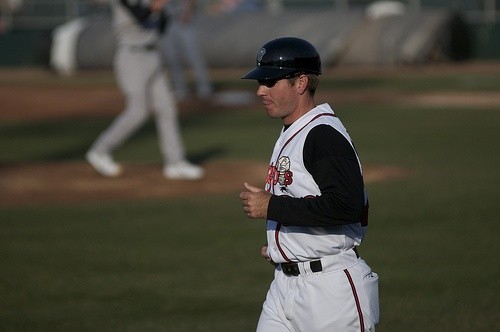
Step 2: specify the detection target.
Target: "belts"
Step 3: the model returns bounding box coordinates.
[281,245,359,276]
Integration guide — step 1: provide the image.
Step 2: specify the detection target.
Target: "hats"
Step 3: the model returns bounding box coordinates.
[240,36,321,81]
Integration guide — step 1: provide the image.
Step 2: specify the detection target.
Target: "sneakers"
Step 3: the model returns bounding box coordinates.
[162,160,205,181]
[86,150,123,178]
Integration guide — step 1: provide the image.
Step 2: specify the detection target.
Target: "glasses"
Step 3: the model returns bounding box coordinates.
[256,80,279,89]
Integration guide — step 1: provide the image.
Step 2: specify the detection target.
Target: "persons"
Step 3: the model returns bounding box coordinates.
[241,37,380,332]
[86,0,206,180]
[160,0,209,99]
